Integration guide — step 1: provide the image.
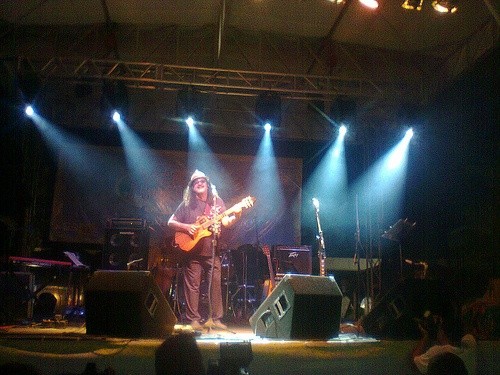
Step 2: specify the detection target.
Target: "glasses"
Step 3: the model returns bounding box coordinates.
[193,179,205,186]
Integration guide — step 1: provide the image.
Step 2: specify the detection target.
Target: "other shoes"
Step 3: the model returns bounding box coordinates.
[190,321,204,330]
[214,319,228,330]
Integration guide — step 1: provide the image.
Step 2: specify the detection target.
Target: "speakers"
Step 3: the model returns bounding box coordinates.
[362,293,427,340]
[272,245,312,275]
[104,228,150,270]
[86,269,178,337]
[249,274,343,341]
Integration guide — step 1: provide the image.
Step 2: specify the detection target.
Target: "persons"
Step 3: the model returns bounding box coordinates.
[167,169,242,332]
[154,334,204,375]
[427,350,468,374]
[412,311,476,374]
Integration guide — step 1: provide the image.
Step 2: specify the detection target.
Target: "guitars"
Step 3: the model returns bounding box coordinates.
[172,194,255,252]
[262,243,280,298]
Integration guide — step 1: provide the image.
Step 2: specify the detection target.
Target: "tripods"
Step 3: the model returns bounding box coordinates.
[190,197,236,335]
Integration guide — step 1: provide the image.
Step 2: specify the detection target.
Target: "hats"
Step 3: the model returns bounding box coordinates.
[191,169,209,182]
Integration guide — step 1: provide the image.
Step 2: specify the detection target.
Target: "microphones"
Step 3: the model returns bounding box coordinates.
[211,185,218,197]
